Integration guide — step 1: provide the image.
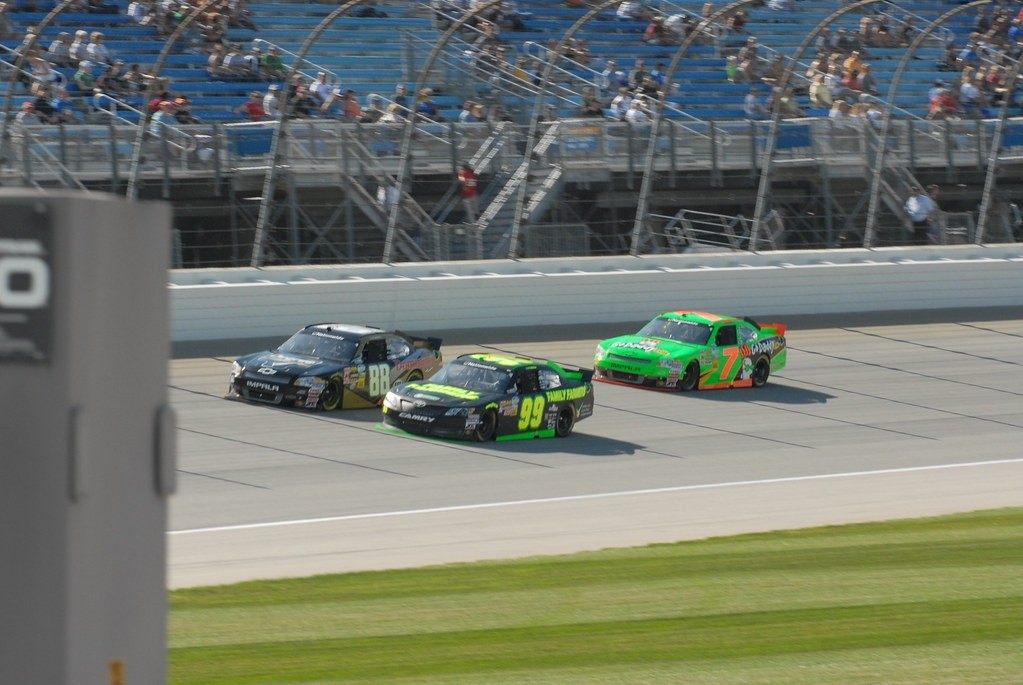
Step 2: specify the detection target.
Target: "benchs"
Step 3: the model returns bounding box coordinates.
[0,0,1023,133]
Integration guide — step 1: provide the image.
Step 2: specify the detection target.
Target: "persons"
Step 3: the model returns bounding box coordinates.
[904,186,934,245]
[0,0,1023,135]
[458,161,481,222]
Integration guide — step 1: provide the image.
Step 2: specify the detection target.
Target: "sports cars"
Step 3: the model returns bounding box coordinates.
[381,352,594,442]
[591,309,787,392]
[226,323,444,412]
